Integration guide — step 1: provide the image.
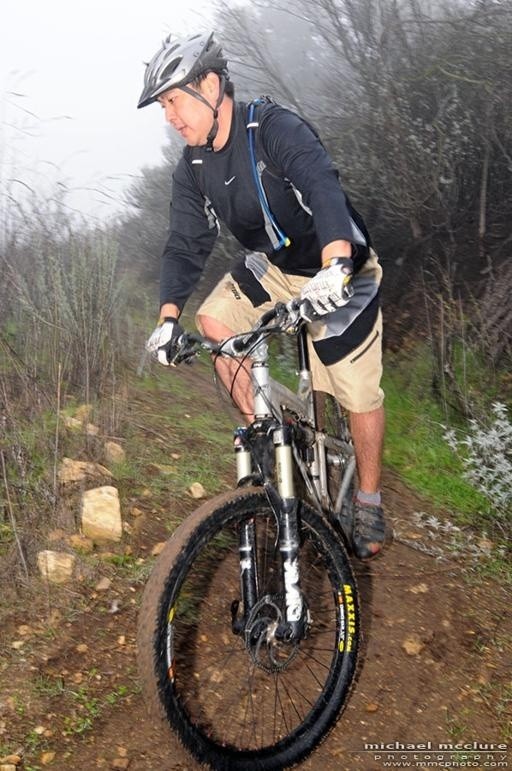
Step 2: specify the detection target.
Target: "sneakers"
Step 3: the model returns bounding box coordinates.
[351,498,387,561]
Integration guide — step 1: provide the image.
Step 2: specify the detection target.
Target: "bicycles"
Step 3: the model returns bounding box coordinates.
[136,286,365,771]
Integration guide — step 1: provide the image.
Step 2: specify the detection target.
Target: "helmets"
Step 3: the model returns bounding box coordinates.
[138,32,227,113]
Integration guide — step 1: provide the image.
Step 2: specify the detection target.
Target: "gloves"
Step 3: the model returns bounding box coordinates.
[148,317,183,365]
[298,257,353,322]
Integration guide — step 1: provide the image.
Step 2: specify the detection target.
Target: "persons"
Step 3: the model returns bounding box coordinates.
[137,31,385,561]
